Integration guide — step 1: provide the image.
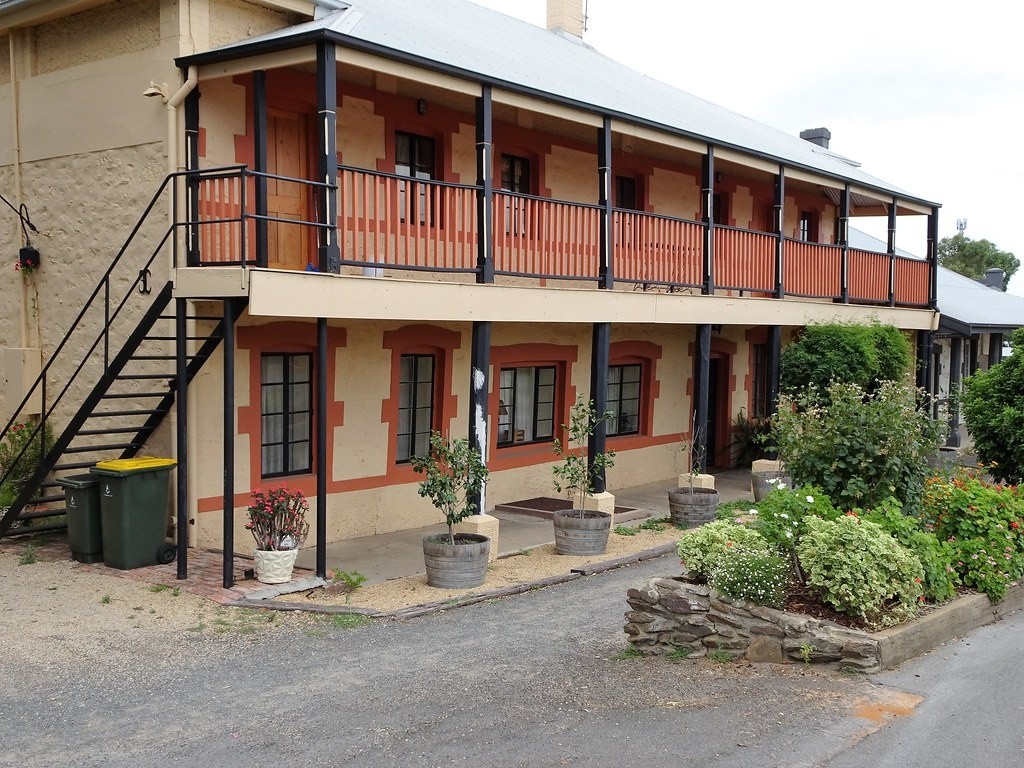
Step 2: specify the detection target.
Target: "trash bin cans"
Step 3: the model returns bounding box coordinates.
[54,472,103,564]
[88,456,176,571]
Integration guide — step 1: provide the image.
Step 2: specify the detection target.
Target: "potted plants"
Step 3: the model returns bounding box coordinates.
[244,481,311,585]
[549,391,616,556]
[408,428,492,589]
[751,400,799,502]
[667,408,719,526]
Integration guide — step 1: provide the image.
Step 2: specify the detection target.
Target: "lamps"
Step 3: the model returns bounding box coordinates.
[416,97,729,183]
